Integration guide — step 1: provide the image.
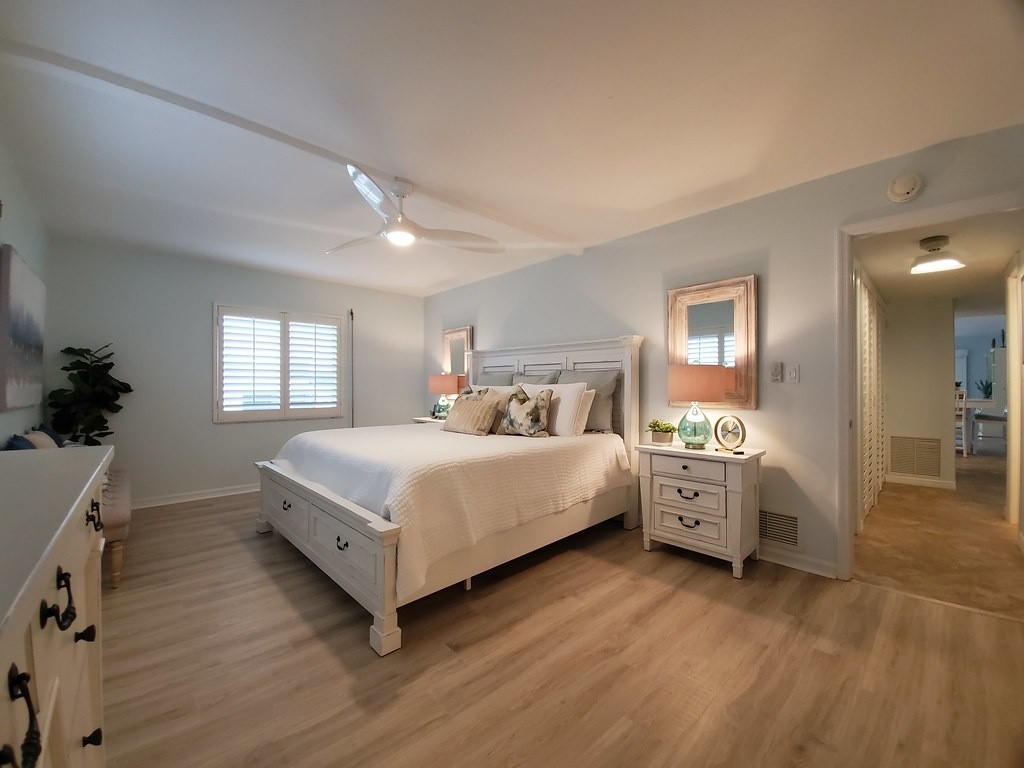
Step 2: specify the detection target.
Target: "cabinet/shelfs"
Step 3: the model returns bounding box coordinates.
[0,444,116,768]
[984,348,1007,400]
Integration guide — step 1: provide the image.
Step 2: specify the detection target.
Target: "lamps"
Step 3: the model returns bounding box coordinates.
[384,224,417,248]
[669,362,726,450]
[910,234,968,275]
[429,374,458,419]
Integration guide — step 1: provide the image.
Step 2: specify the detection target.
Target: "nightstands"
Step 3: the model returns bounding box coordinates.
[633,443,766,577]
[411,415,446,424]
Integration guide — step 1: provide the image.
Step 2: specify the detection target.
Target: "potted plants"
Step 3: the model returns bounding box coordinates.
[955,381,963,398]
[975,379,994,400]
[645,419,677,447]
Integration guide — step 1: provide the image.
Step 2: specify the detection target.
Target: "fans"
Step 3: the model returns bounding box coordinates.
[329,159,504,255]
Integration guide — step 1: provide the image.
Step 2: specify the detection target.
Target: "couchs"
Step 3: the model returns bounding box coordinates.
[3,423,134,589]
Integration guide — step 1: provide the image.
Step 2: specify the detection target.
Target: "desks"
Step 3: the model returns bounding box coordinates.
[954,399,995,454]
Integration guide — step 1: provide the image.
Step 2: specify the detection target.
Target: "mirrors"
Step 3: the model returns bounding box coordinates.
[666,275,760,410]
[441,325,473,399]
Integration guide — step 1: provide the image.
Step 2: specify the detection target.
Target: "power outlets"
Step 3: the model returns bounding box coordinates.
[768,363,783,383]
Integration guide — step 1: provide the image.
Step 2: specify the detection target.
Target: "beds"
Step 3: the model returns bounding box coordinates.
[253,334,644,656]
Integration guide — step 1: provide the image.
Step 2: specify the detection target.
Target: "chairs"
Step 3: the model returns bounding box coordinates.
[973,408,1007,454]
[955,390,967,457]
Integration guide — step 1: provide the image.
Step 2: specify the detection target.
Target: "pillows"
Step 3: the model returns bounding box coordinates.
[556,369,621,433]
[515,381,587,436]
[574,389,596,436]
[475,370,514,385]
[470,383,513,395]
[454,385,489,402]
[6,424,64,449]
[483,390,511,434]
[441,398,501,436]
[496,384,553,437]
[513,370,561,384]
